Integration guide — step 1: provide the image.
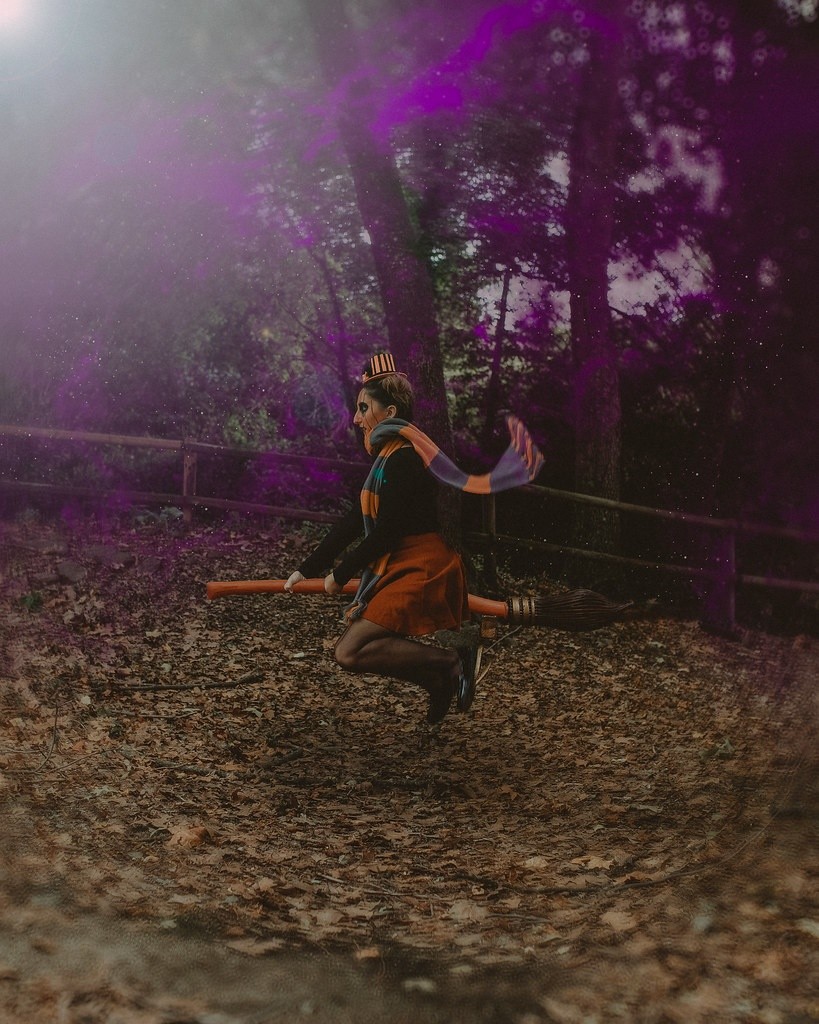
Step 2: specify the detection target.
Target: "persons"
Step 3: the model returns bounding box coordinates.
[284,373,476,725]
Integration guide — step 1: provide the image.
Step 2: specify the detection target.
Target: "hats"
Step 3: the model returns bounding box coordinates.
[362,351,409,386]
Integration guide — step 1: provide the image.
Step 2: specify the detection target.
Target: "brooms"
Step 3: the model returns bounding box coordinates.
[204,580,654,634]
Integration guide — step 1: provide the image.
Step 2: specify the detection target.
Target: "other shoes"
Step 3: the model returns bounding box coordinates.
[456,645,477,712]
[426,674,455,726]
